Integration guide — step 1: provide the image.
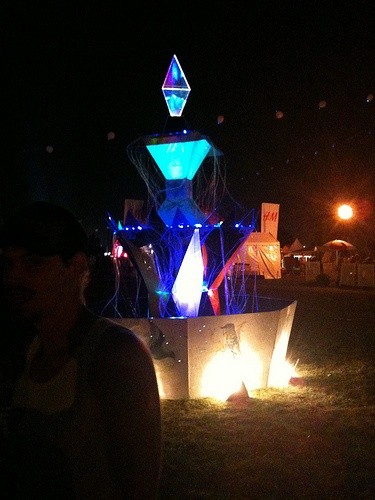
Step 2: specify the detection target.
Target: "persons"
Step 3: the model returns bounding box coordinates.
[284,253,375,269]
[1,201,162,500]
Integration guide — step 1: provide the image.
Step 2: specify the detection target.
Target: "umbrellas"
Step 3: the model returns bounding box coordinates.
[320,238,356,264]
